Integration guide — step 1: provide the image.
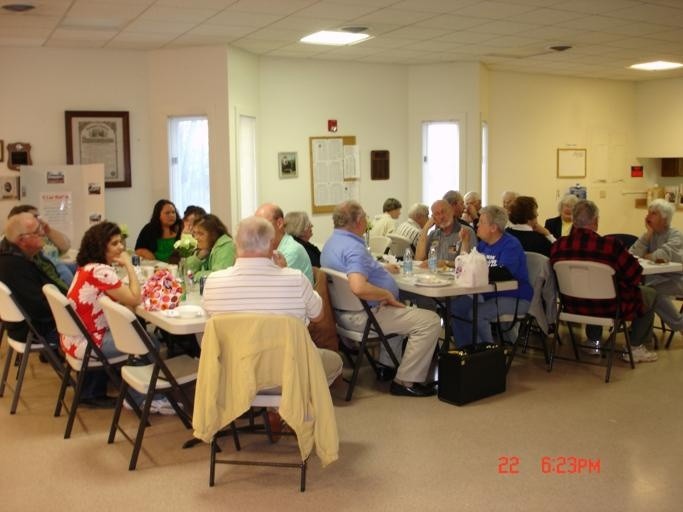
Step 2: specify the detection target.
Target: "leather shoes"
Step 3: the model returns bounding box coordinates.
[263,411,282,444]
[377,367,396,381]
[390,380,438,396]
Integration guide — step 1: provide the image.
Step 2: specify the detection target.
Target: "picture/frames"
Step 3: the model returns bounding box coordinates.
[64,109,132,188]
[557,148,587,179]
[277,151,299,180]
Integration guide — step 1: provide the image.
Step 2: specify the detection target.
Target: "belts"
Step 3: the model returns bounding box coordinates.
[334,305,375,312]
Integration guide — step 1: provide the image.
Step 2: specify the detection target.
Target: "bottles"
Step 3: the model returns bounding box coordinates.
[427,245,438,274]
[177,257,187,306]
[646,184,665,207]
[402,247,413,278]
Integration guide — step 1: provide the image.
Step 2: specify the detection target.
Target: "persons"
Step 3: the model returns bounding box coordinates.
[626,197,683,352]
[253,200,316,287]
[363,189,560,365]
[133,197,186,343]
[198,215,345,446]
[543,192,584,242]
[56,220,186,416]
[6,203,72,365]
[172,206,207,262]
[283,210,323,270]
[317,197,442,397]
[547,198,659,365]
[1,211,120,412]
[159,212,238,359]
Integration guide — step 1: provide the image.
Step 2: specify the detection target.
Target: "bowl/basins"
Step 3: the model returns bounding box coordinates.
[176,305,200,319]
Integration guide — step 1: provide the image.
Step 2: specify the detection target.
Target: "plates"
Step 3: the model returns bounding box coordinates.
[399,260,422,267]
[412,273,449,286]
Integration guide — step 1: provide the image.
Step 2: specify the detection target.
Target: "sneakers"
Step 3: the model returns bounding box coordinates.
[77,395,184,415]
[622,344,658,363]
[40,350,65,362]
[579,339,601,356]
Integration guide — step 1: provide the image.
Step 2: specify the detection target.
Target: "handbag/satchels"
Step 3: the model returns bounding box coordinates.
[488,267,512,281]
[438,342,505,406]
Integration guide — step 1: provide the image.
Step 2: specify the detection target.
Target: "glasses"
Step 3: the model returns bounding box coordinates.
[19,224,42,236]
